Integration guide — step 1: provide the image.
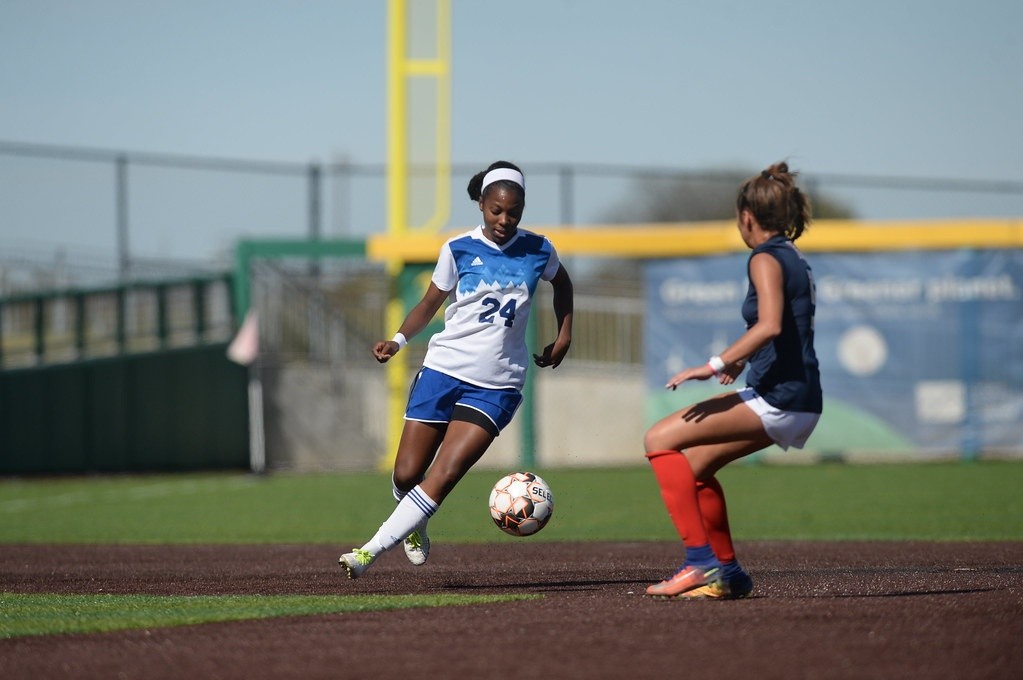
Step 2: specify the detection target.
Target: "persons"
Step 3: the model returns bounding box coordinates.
[339,160,573,579]
[643,162,823,600]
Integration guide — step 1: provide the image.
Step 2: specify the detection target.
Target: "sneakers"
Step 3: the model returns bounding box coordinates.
[678,572,752,599]
[646,556,721,599]
[339,548,376,580]
[404,517,430,566]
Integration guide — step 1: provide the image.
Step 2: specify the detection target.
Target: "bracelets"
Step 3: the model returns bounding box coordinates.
[708,356,725,375]
[392,333,407,351]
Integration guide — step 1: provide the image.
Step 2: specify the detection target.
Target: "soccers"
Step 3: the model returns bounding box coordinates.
[488,471,554,537]
